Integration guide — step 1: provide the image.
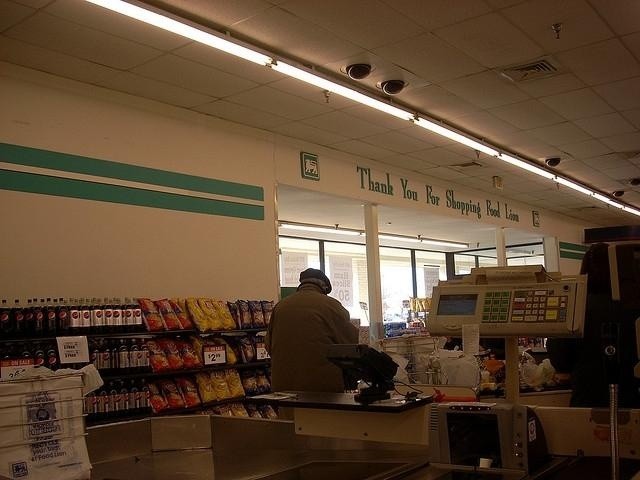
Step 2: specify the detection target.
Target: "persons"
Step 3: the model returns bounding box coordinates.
[264,268,359,393]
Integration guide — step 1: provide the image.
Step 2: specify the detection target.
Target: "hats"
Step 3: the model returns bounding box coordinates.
[300,268,332,294]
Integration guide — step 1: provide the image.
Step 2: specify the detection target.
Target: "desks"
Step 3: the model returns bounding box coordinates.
[81,414,427,480]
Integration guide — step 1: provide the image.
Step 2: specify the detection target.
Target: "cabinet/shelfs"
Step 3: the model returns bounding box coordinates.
[1,297,278,422]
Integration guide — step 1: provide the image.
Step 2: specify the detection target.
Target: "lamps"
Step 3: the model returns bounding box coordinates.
[83,0,639,218]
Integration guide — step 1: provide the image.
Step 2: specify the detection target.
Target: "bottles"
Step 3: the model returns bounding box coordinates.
[3,297,150,421]
